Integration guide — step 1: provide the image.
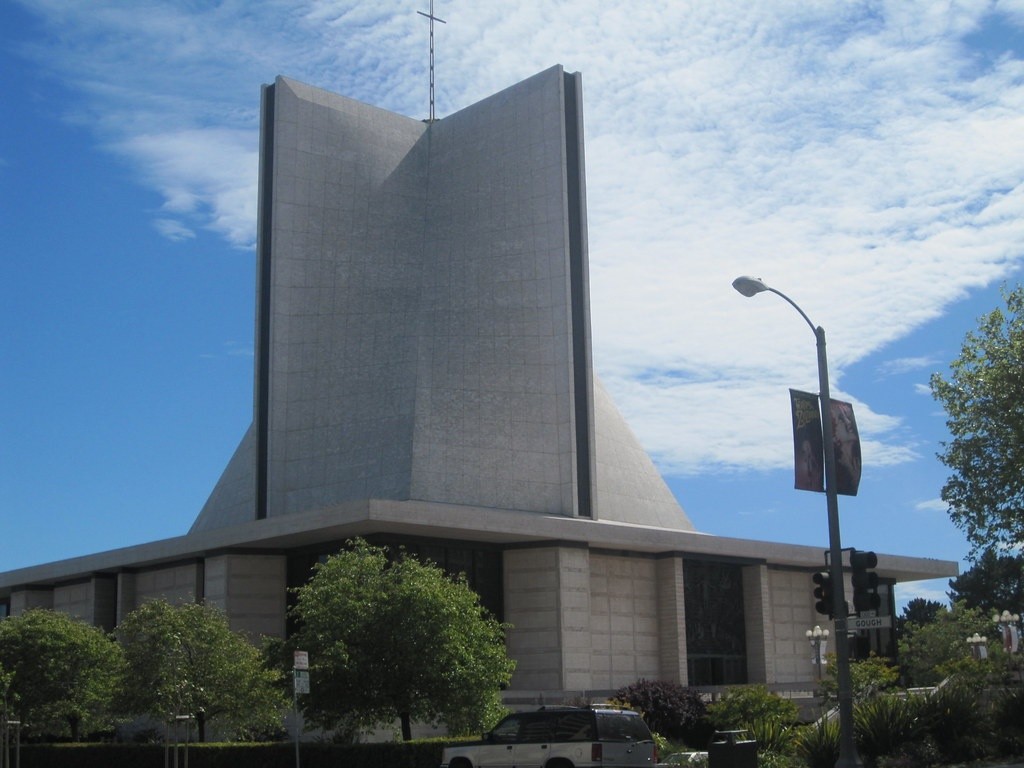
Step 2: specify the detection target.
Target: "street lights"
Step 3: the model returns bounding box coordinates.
[966,632,987,660]
[805,625,830,683]
[730,275,863,767]
[992,609,1020,664]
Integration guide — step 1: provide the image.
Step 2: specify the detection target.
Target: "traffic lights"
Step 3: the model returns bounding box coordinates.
[848,549,880,612]
[811,569,835,619]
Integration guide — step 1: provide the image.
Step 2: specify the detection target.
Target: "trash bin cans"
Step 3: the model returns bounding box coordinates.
[708,730,757,768]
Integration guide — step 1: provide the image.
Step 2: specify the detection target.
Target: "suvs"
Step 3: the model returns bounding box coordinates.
[441,704,658,768]
